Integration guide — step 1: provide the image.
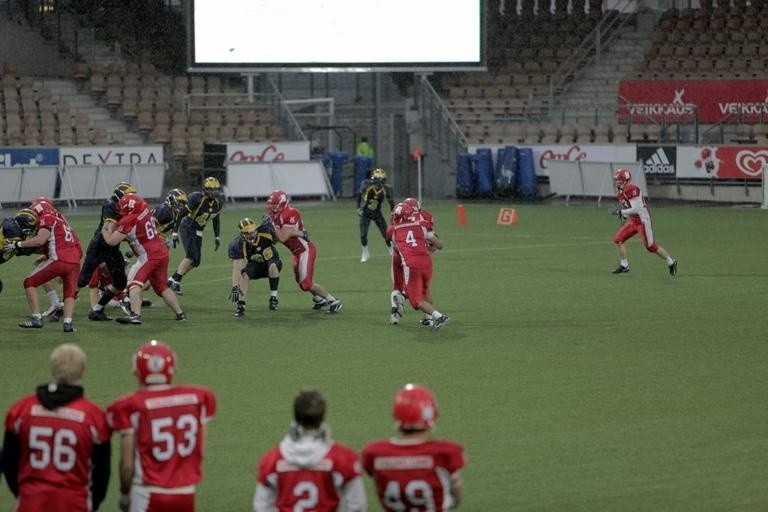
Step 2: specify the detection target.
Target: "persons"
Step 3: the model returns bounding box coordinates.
[263,190,343,316]
[356,135,373,159]
[106,339,215,512]
[3,343,111,512]
[613,168,678,276]
[360,383,467,512]
[227,217,311,317]
[1,176,224,332]
[354,166,450,332]
[251,388,369,512]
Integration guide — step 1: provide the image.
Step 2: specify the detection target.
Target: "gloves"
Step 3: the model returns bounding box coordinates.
[214,238,220,251]
[171,235,180,249]
[228,286,243,302]
[304,230,311,242]
[608,205,620,216]
[2,238,19,254]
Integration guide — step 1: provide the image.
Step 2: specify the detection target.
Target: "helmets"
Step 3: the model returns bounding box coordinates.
[238,218,257,244]
[118,193,144,218]
[131,338,178,386]
[201,176,221,200]
[164,188,192,217]
[403,197,421,213]
[266,190,289,215]
[12,208,40,239]
[390,202,413,224]
[110,182,137,201]
[392,382,440,432]
[34,200,53,217]
[29,197,47,206]
[371,167,388,190]
[613,169,631,190]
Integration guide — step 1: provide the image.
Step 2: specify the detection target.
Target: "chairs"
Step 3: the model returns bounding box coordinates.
[465,123,690,144]
[630,5,768,80]
[440,8,613,119]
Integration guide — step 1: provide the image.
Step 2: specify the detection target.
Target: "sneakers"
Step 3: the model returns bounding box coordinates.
[612,264,629,273]
[268,297,279,312]
[142,299,152,307]
[311,298,328,310]
[42,301,64,317]
[360,246,370,262]
[63,322,73,333]
[176,312,186,321]
[432,314,450,331]
[325,299,343,315]
[390,313,399,325]
[397,304,405,317]
[91,311,113,321]
[417,318,434,327]
[120,301,132,316]
[88,309,95,319]
[18,317,44,328]
[669,258,677,277]
[116,313,143,325]
[47,306,65,322]
[172,285,182,295]
[166,278,174,289]
[232,301,246,319]
